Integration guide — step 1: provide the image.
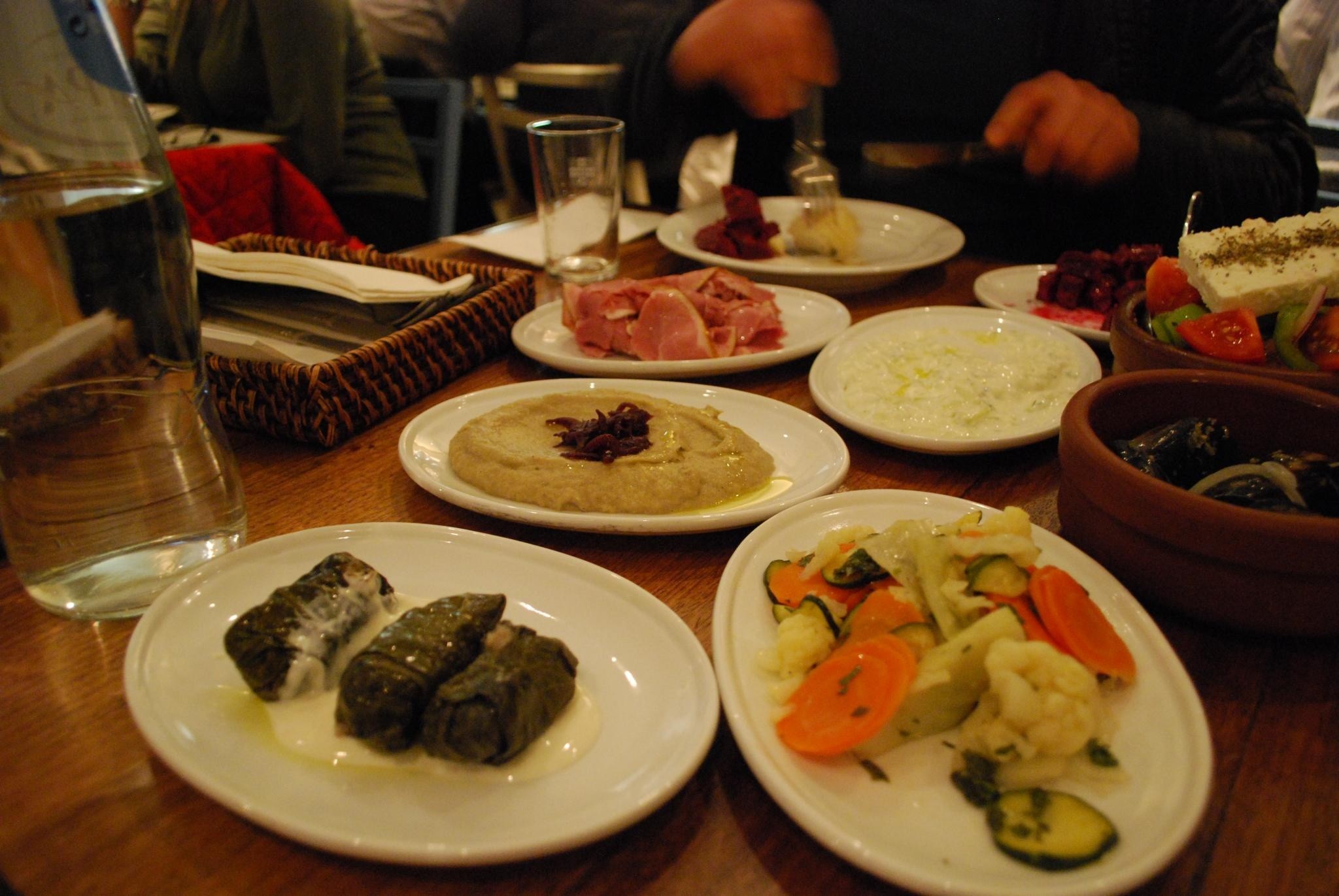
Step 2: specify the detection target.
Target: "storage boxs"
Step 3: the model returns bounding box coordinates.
[205,232,536,449]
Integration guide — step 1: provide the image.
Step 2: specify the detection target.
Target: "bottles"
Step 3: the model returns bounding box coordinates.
[0,0,247,617]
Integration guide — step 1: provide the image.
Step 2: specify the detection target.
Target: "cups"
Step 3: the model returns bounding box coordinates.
[525,118,625,279]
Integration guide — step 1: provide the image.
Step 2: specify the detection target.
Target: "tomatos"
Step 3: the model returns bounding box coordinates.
[1142,256,1339,372]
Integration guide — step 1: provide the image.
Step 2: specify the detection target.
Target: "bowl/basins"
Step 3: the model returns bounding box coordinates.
[1110,293,1339,404]
[1058,369,1338,645]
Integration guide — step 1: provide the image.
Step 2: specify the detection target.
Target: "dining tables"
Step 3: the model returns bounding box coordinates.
[0,204,1339,896]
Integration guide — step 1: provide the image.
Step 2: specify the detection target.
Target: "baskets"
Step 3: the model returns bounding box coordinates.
[207,233,536,457]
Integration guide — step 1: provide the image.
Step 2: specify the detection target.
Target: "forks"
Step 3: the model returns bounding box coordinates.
[375,291,454,331]
[452,280,495,306]
[793,82,841,228]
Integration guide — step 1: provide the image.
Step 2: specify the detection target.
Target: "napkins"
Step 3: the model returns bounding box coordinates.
[171,194,670,367]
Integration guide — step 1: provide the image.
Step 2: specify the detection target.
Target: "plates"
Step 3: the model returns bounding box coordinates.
[975,263,1110,344]
[657,197,965,294]
[398,375,848,537]
[512,281,850,381]
[712,488,1214,895]
[122,523,721,866]
[809,306,1104,454]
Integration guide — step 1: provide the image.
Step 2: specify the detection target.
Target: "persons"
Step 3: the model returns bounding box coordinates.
[103,0,1339,259]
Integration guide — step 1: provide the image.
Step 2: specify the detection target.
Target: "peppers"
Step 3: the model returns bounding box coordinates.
[1153,302,1207,346]
[1276,306,1327,371]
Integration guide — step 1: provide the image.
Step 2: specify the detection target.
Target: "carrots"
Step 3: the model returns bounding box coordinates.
[770,543,1136,757]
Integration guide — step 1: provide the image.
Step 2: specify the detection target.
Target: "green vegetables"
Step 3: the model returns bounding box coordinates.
[826,547,1119,840]
